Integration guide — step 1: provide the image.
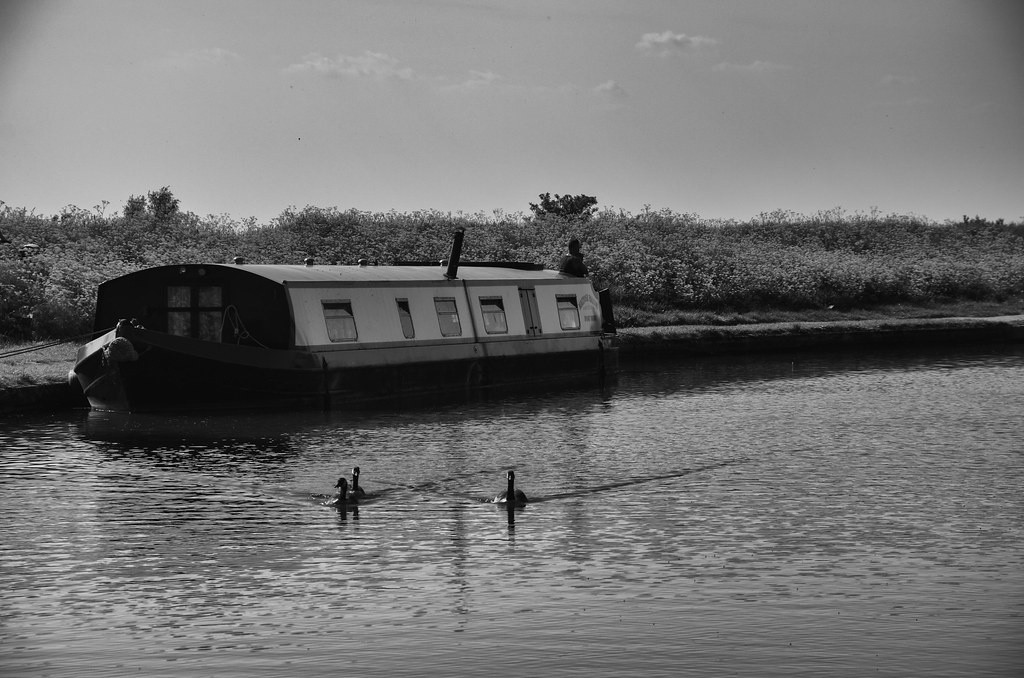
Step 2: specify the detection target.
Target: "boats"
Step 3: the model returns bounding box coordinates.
[73,230,615,415]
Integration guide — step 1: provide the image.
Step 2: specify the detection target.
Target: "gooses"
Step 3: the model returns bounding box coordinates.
[325,466,366,505]
[493,470,528,503]
